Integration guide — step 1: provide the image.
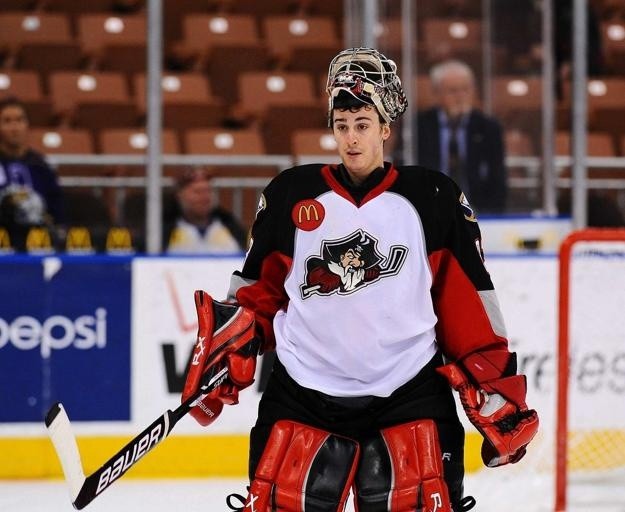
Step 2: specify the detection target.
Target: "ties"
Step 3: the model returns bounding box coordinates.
[446,118,462,173]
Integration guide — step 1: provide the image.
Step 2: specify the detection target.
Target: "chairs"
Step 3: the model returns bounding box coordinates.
[0,0,625,230]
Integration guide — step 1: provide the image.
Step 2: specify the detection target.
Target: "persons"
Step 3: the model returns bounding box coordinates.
[134,168,252,255]
[0,94,109,254]
[394,60,510,214]
[181,46,543,511]
[487,1,607,81]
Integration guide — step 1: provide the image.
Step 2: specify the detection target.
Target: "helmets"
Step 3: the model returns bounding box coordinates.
[322,47,412,126]
